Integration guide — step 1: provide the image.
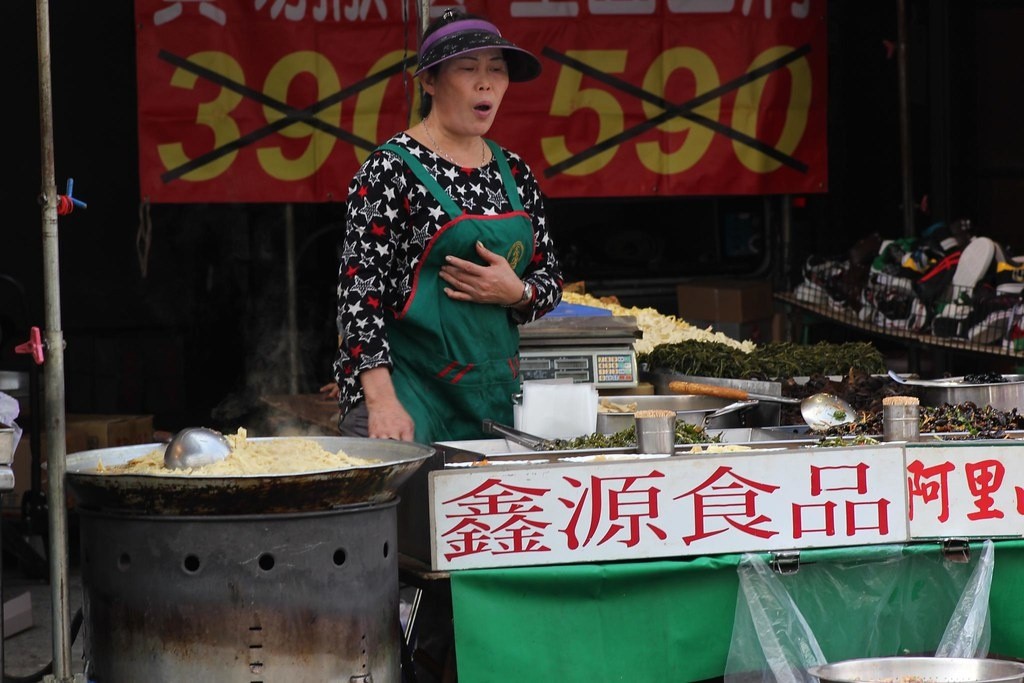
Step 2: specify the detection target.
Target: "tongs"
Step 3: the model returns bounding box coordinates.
[482,418,568,452]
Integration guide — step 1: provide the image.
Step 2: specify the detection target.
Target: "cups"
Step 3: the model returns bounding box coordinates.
[882,404,920,442]
[635,416,676,456]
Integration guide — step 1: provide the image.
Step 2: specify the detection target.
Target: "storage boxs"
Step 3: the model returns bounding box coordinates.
[66,413,152,453]
[676,280,771,325]
[0,428,89,509]
[684,319,772,347]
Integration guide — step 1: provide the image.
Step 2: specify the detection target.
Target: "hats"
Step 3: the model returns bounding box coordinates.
[411,17,542,82]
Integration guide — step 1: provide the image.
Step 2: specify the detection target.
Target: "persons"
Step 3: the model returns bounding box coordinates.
[338,14,565,560]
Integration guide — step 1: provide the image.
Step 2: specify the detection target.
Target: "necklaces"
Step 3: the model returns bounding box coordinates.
[422,117,486,168]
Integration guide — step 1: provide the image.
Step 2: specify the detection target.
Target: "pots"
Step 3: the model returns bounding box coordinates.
[40,434,438,514]
[888,370,1024,419]
[597,393,758,436]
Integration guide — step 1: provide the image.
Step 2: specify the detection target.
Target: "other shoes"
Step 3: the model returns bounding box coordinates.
[797,229,1024,352]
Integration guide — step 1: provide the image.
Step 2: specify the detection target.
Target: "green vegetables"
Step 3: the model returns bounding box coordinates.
[632,337,884,381]
[551,417,724,453]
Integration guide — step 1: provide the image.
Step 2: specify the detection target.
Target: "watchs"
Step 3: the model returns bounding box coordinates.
[501,280,532,308]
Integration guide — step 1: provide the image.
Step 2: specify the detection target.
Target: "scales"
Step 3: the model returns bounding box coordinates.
[518,315,642,389]
